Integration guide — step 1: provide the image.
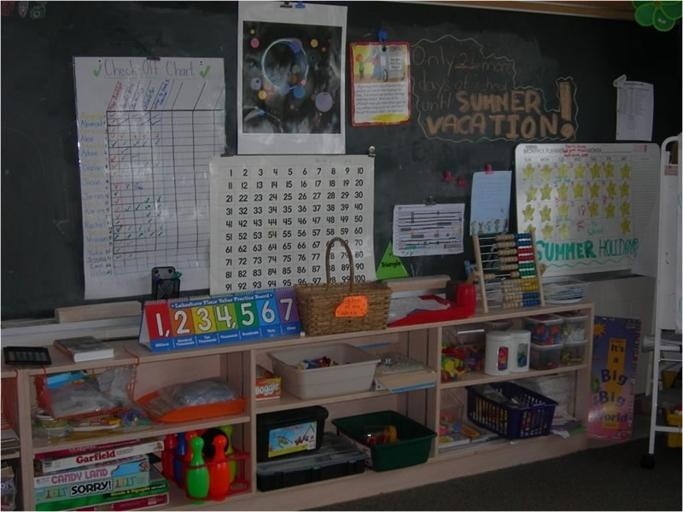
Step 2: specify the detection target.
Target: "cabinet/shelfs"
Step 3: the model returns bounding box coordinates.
[1,298,594,511]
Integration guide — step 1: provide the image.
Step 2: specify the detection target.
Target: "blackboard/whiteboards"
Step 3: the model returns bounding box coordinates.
[0,1,683,330]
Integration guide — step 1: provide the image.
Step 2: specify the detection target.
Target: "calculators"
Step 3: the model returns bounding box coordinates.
[3,346,52,366]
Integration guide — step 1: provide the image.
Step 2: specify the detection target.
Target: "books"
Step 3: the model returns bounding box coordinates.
[436,418,490,455]
[52,336,114,363]
[0,408,21,450]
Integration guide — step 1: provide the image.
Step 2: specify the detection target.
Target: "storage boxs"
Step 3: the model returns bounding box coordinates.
[266,341,382,400]
[255,405,438,492]
[525,312,590,369]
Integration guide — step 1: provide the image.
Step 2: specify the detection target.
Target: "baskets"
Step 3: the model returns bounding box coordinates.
[293,236,391,337]
[463,381,559,440]
